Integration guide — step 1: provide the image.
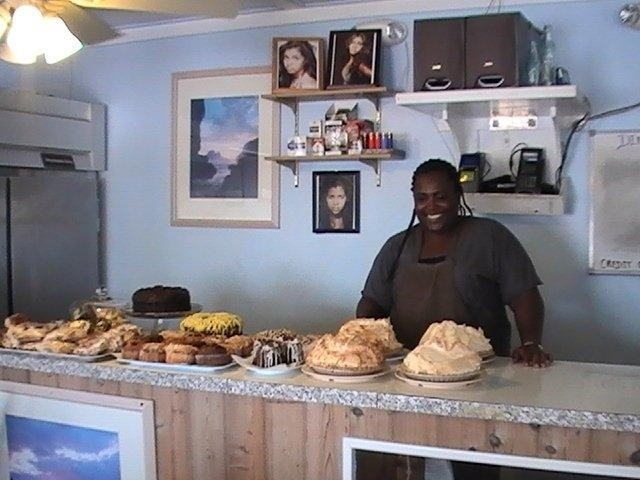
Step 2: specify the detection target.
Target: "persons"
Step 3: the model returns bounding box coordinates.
[335,31,375,83]
[276,38,317,88]
[355,156,556,369]
[317,173,355,230]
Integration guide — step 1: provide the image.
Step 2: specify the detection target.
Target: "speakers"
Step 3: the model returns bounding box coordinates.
[466,12,547,89]
[413,17,466,92]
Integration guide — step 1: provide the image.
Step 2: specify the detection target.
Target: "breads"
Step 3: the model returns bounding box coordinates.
[253,317,496,380]
[0,310,253,366]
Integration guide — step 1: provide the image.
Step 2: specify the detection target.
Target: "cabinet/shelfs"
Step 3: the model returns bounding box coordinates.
[395,83,578,217]
[263,81,404,188]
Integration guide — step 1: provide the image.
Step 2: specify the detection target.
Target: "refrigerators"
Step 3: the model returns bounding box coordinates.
[0,170,99,325]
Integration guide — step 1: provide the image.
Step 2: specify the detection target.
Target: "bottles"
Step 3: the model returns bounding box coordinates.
[363,131,393,149]
[527,41,542,86]
[539,25,557,85]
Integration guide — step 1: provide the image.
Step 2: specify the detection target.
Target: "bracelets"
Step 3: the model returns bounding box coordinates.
[521,337,547,352]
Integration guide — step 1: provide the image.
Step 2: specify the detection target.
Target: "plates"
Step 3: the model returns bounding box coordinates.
[229,349,498,390]
[118,347,237,372]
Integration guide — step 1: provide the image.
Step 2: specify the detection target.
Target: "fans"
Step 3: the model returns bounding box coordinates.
[0,1,241,46]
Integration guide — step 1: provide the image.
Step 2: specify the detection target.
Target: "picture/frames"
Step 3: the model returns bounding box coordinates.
[271,34,325,90]
[311,168,358,232]
[325,28,381,89]
[0,378,163,480]
[169,61,281,230]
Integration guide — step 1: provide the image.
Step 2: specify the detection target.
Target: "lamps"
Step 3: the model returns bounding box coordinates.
[0,0,84,66]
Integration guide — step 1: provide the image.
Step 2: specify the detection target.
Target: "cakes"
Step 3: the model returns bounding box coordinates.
[130,285,193,313]
